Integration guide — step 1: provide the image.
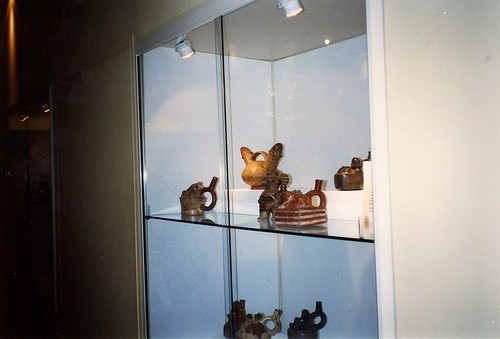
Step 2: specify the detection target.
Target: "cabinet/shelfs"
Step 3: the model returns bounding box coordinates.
[130,0,396,339]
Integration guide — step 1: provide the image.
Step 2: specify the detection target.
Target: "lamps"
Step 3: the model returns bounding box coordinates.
[19,112,30,122]
[280,0,302,17]
[175,40,195,59]
[39,102,49,113]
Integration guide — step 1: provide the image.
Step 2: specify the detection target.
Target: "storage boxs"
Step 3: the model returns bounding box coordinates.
[229,189,264,216]
[321,191,363,220]
